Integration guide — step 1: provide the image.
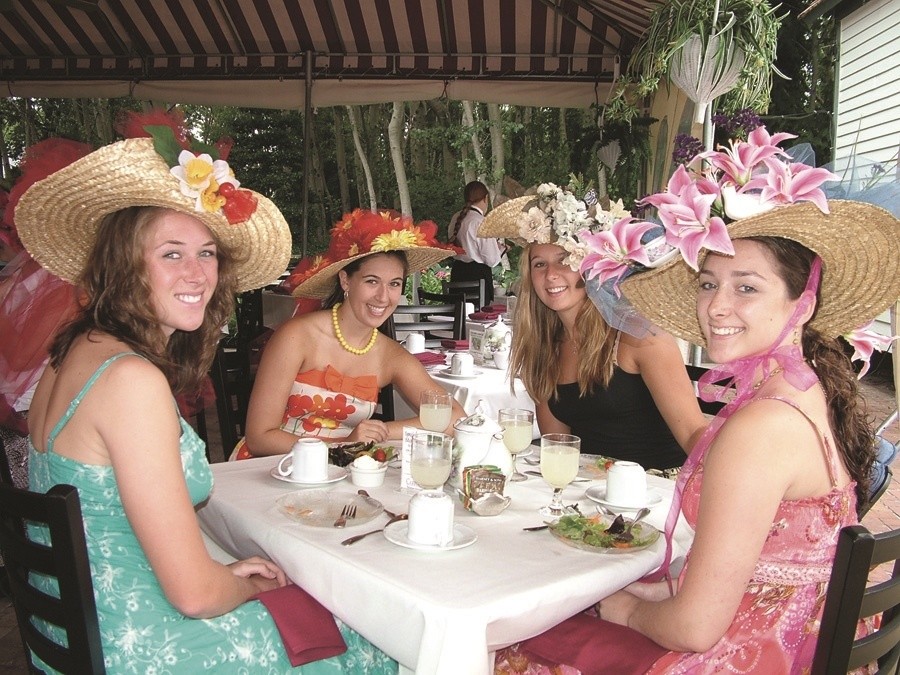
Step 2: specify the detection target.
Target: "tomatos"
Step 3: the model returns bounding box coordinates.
[605,462,612,469]
[374,449,386,462]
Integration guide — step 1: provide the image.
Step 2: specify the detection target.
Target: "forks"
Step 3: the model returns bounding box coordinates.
[334,504,357,528]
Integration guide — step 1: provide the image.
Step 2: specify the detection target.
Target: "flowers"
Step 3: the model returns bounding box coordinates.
[142,124,259,225]
[281,209,466,289]
[517,108,843,301]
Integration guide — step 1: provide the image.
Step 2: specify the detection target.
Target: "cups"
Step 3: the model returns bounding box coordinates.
[410,431,452,490]
[407,489,455,545]
[420,389,452,432]
[494,351,510,370]
[277,437,389,489]
[605,461,649,506]
[399,333,425,355]
[451,353,474,376]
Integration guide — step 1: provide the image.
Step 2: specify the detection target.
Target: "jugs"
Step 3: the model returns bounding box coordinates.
[480,314,513,359]
[446,399,514,493]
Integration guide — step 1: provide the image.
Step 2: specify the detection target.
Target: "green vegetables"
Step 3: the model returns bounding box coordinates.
[368,443,394,460]
[553,515,655,547]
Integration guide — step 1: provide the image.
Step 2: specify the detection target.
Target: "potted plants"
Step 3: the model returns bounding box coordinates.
[573,116,660,178]
[604,0,792,127]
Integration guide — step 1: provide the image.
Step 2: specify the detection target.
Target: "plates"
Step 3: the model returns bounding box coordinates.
[576,454,620,480]
[439,369,483,379]
[548,483,667,554]
[384,520,478,550]
[269,461,384,530]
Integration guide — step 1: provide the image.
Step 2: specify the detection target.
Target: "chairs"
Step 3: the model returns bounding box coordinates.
[381,301,463,349]
[441,279,485,312]
[194,289,270,463]
[812,526,900,675]
[417,287,466,339]
[0,439,106,675]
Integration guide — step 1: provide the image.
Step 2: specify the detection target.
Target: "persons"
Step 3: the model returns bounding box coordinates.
[492,128,900,675]
[0,105,714,674]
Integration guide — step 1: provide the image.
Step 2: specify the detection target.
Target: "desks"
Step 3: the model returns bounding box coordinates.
[261,290,295,330]
[195,445,695,675]
[374,349,541,441]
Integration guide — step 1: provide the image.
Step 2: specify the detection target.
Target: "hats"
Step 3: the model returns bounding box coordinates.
[286,210,466,299]
[476,172,632,254]
[13,113,293,296]
[580,127,900,353]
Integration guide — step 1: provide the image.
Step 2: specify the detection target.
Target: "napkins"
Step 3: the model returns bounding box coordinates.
[441,340,469,350]
[413,351,448,365]
[467,304,507,320]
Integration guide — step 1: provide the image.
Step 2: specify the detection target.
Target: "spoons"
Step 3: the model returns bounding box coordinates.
[523,457,540,466]
[342,513,408,546]
[596,505,666,536]
[613,508,650,544]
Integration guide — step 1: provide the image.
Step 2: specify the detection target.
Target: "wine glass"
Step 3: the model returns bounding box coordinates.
[537,433,581,518]
[499,408,534,482]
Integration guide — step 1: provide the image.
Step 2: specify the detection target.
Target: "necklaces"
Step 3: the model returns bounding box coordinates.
[331,303,378,354]
[748,356,806,396]
